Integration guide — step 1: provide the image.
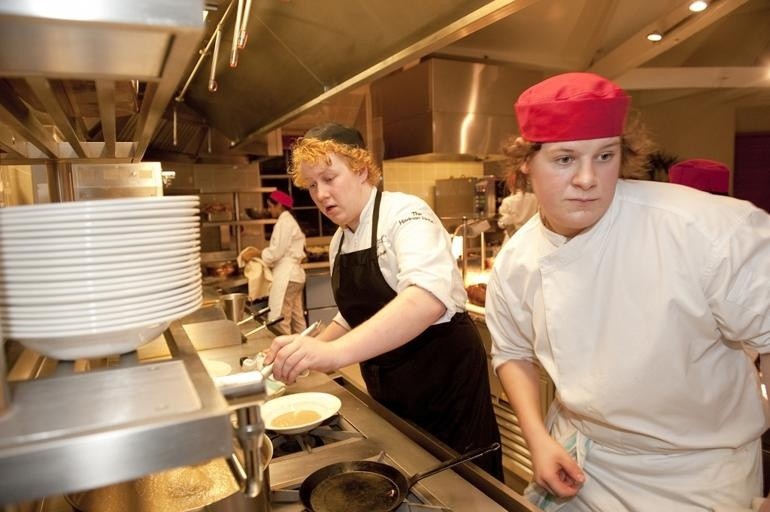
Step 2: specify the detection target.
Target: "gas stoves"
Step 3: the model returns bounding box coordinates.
[231,380,452,512]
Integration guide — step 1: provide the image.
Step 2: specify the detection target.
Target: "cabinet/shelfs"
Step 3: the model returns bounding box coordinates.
[164,186,278,275]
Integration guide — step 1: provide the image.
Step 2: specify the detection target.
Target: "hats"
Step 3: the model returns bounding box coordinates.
[270,191,293,209]
[670,159,730,194]
[305,124,366,149]
[513,72,632,142]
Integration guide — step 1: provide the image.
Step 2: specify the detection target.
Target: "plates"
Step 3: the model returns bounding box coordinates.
[0,196,203,362]
[257,393,339,433]
[208,360,233,378]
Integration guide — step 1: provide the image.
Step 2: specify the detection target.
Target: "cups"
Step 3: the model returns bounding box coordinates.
[222,293,248,321]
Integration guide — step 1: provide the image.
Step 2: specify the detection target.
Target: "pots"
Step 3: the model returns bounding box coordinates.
[303,445,505,512]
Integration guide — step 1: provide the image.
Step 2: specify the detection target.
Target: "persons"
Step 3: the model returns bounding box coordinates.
[241,191,308,337]
[495,166,536,246]
[482,71,770,512]
[260,123,505,482]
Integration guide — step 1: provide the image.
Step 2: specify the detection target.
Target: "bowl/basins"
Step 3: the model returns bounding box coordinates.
[246,207,272,219]
